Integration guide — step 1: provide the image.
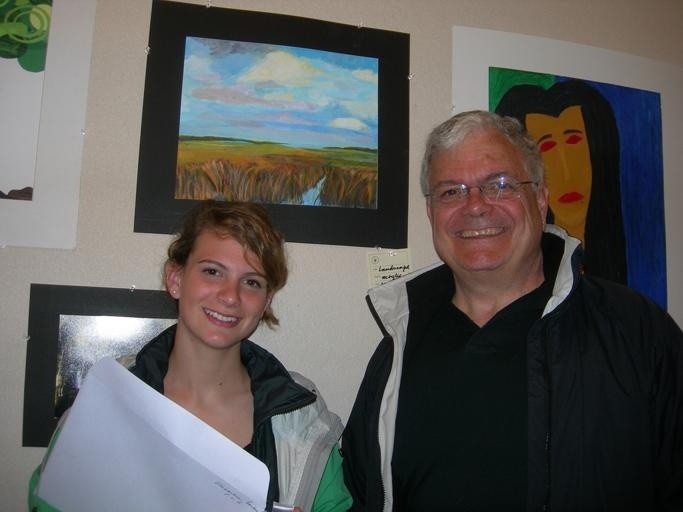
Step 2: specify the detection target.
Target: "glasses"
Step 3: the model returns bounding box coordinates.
[423,173,538,210]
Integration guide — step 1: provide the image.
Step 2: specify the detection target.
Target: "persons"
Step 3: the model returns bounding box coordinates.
[336,104,683,512]
[25,200,356,512]
